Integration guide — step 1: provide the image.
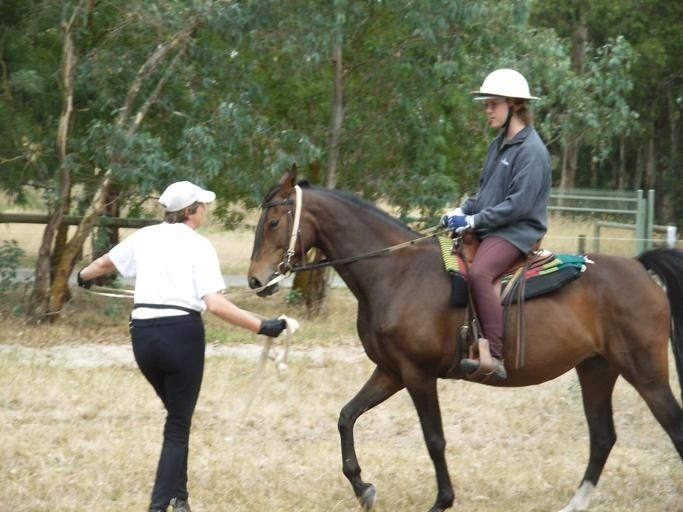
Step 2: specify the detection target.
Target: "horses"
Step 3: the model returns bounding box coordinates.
[246,162,683,512]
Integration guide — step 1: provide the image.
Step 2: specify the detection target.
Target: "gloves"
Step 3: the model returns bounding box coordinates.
[257,317,287,337]
[77,268,94,289]
[441,208,476,233]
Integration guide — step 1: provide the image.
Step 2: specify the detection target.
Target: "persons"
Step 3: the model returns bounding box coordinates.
[74,181,289,511]
[440,69,552,382]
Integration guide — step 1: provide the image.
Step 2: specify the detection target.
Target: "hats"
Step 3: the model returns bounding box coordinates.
[157,181,216,212]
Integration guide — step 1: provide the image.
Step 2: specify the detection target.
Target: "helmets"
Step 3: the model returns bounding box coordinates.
[470,68,541,101]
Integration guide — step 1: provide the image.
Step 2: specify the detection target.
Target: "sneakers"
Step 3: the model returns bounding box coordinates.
[460,356,507,383]
[172,497,191,512]
